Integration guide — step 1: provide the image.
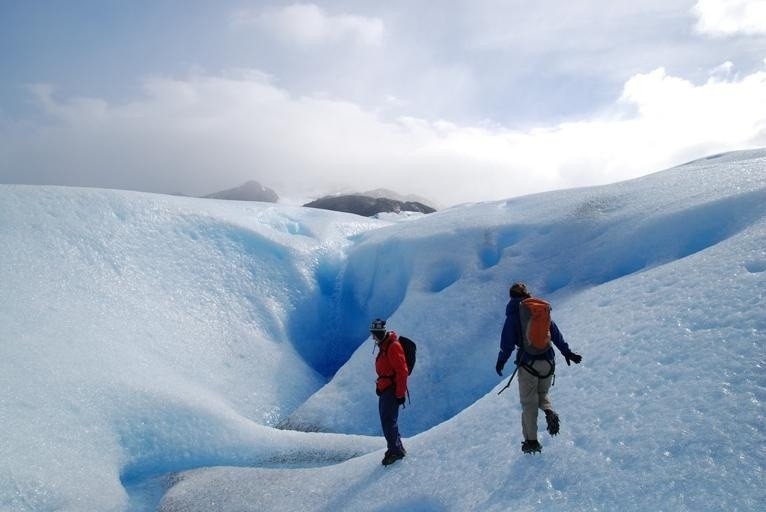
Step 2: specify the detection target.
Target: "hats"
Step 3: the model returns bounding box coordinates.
[369,318,387,332]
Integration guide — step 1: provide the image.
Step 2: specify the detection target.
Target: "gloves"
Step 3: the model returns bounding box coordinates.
[563,350,583,366]
[496,360,506,377]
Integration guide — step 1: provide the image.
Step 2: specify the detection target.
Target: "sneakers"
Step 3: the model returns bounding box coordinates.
[382,443,407,465]
[546,412,560,435]
[522,439,542,452]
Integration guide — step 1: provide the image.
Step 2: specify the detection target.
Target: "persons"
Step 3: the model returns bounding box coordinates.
[367,320,416,465]
[497,283,584,455]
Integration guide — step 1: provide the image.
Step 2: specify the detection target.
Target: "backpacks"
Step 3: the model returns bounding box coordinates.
[519,297,552,356]
[375,336,417,379]
